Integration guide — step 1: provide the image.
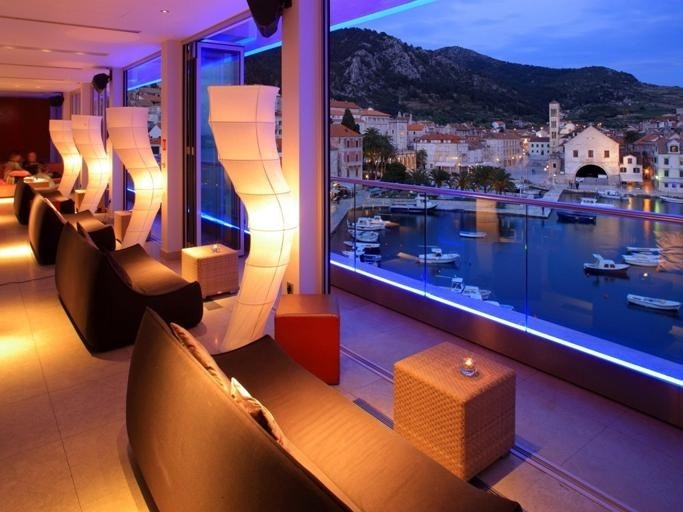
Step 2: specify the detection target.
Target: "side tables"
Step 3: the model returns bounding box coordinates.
[393,340,515,482]
[181,242,239,299]
[112,211,151,244]
[74,190,104,211]
[274,295,340,386]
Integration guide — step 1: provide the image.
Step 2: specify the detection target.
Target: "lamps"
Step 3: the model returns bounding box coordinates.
[106,107,165,248]
[72,114,112,215]
[207,86,294,352]
[50,119,84,198]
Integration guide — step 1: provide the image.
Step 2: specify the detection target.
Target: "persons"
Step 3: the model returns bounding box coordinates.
[4,151,39,184]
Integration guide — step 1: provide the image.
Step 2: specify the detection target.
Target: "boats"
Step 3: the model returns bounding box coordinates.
[658,194,683,204]
[459,231,488,238]
[451,277,514,311]
[556,210,597,226]
[416,247,461,265]
[627,293,681,311]
[597,189,625,199]
[583,246,660,276]
[388,190,440,214]
[576,197,598,204]
[341,213,401,267]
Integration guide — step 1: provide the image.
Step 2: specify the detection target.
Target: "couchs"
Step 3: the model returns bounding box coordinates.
[125,307,520,508]
[28,192,116,265]
[15,179,63,223]
[54,221,203,353]
